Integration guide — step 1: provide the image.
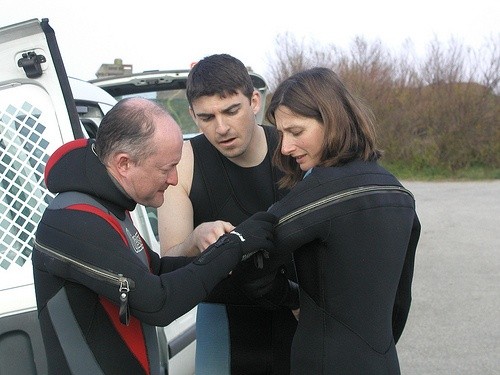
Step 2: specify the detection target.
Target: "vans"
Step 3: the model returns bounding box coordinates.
[0,14,274,375]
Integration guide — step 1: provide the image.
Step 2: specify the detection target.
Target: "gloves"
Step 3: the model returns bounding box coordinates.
[228,213,279,263]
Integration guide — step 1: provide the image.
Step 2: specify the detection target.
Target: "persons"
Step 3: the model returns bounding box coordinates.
[32,97,279,375]
[157,54,306,375]
[265,67,420,375]
